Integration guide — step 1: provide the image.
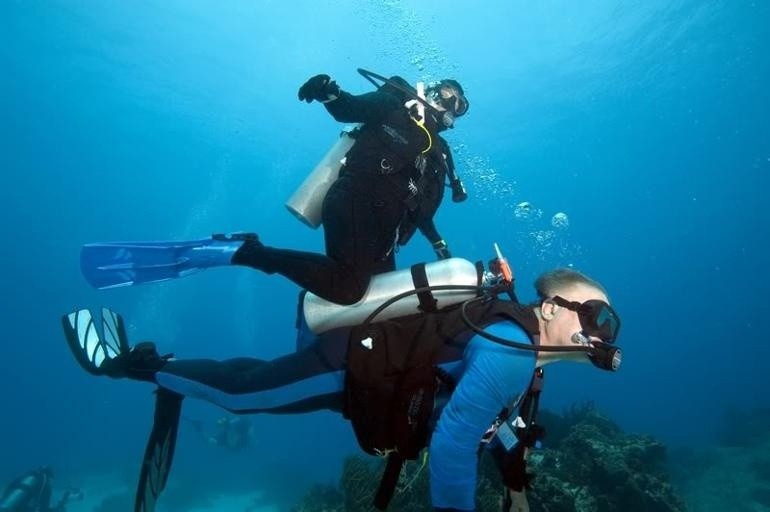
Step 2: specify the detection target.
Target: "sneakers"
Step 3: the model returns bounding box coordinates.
[186,233,258,269]
[104,341,163,381]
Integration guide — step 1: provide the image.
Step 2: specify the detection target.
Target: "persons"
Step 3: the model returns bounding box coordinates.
[176,67,472,307]
[61,242,623,511]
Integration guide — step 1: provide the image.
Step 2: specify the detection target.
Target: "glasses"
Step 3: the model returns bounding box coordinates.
[438,80,469,117]
[555,296,621,344]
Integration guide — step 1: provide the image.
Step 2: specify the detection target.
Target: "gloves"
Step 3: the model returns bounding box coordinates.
[502,481,530,511]
[432,240,450,259]
[298,74,341,104]
